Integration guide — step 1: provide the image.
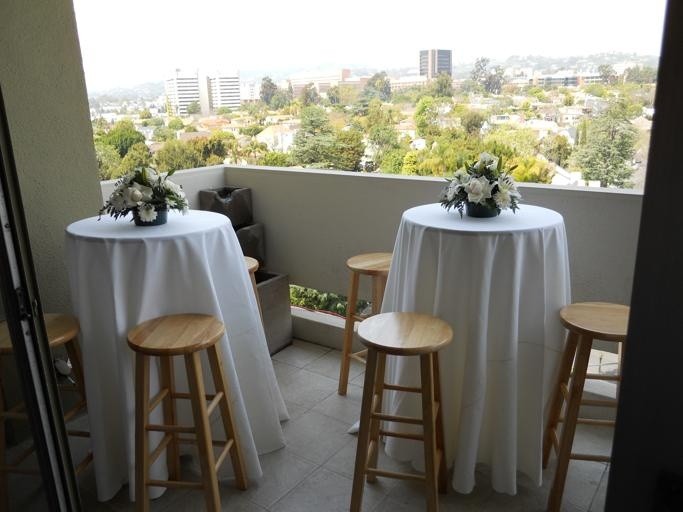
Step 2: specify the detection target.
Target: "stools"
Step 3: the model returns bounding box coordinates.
[126,313,249,512]
[0,311,94,512]
[338,251,392,396]
[542,301,630,512]
[243,256,264,328]
[349,312,454,512]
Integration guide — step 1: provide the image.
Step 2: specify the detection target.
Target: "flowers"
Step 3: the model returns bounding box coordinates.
[289,286,367,317]
[438,147,524,219]
[97,166,192,223]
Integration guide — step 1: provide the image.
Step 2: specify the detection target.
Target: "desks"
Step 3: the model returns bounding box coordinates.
[64,209,290,503]
[346,202,572,498]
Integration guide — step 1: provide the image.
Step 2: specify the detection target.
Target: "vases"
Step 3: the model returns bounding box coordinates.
[132,206,166,226]
[465,198,498,218]
